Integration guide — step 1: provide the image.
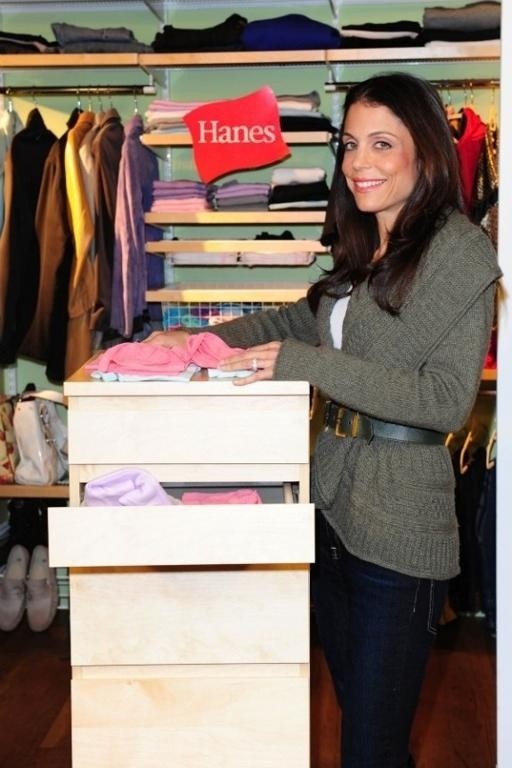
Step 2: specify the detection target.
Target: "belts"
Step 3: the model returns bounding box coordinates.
[320,401,447,444]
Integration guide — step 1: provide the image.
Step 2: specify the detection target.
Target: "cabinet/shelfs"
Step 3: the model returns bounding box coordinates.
[322,1,500,642]
[137,0,338,357]
[0,2,151,630]
[46,344,316,767]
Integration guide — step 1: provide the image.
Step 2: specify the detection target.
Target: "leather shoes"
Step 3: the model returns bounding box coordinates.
[27,543,59,631]
[0,545,28,631]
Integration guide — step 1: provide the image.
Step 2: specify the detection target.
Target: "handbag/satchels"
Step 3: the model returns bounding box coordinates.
[14,390,67,486]
[0,395,18,484]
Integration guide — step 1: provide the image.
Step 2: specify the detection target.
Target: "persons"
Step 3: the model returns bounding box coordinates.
[139,70,498,768]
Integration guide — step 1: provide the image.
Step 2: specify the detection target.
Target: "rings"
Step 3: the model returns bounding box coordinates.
[251,359,258,371]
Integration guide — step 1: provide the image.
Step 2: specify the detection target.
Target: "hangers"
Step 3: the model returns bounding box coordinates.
[435,390,497,474]
[421,73,501,131]
[3,80,157,130]
